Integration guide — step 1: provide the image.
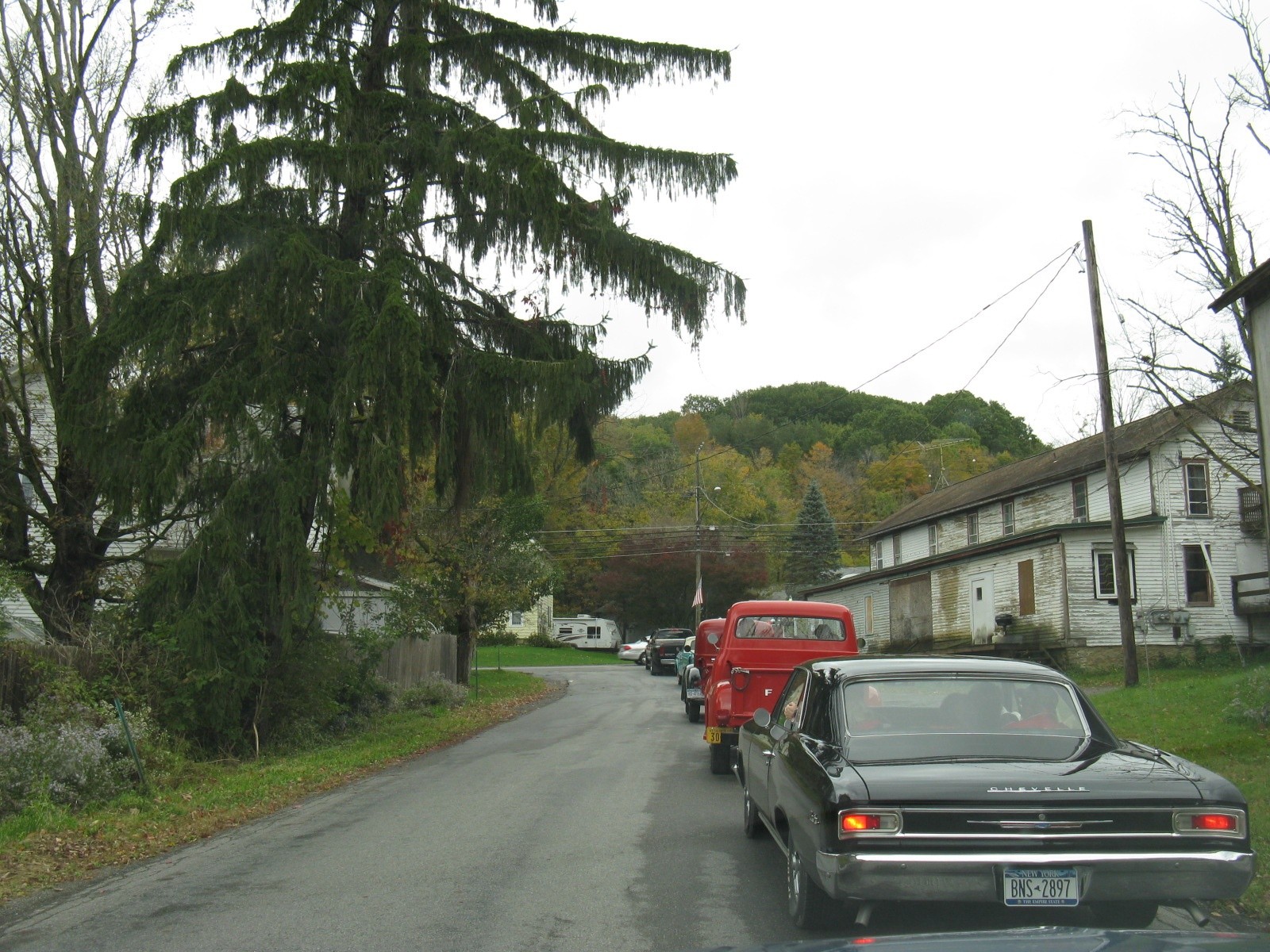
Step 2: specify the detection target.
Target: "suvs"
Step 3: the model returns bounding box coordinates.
[641,628,696,675]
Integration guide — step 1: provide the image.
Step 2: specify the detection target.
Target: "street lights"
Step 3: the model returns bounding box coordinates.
[695,486,721,634]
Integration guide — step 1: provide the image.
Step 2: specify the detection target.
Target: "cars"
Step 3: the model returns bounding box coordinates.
[616,635,678,666]
[733,654,1261,930]
[676,601,881,774]
